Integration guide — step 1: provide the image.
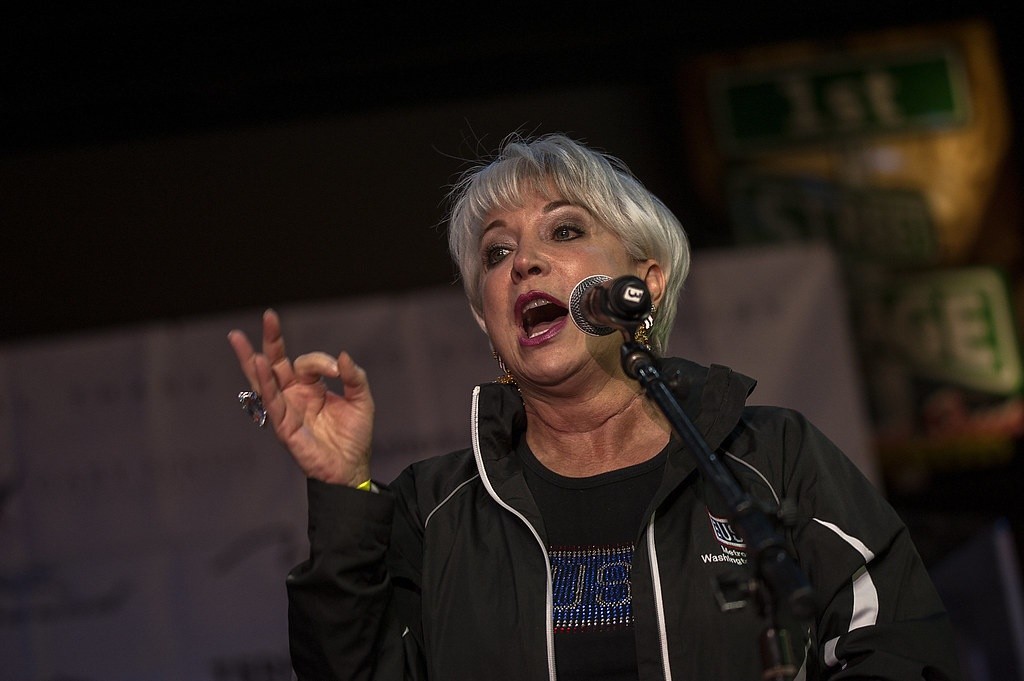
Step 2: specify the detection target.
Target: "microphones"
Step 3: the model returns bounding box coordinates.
[568,274,651,337]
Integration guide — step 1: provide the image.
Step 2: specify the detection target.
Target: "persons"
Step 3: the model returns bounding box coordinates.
[226,135,961,680]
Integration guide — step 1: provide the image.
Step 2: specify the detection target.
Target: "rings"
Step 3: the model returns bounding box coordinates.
[238,391,267,427]
